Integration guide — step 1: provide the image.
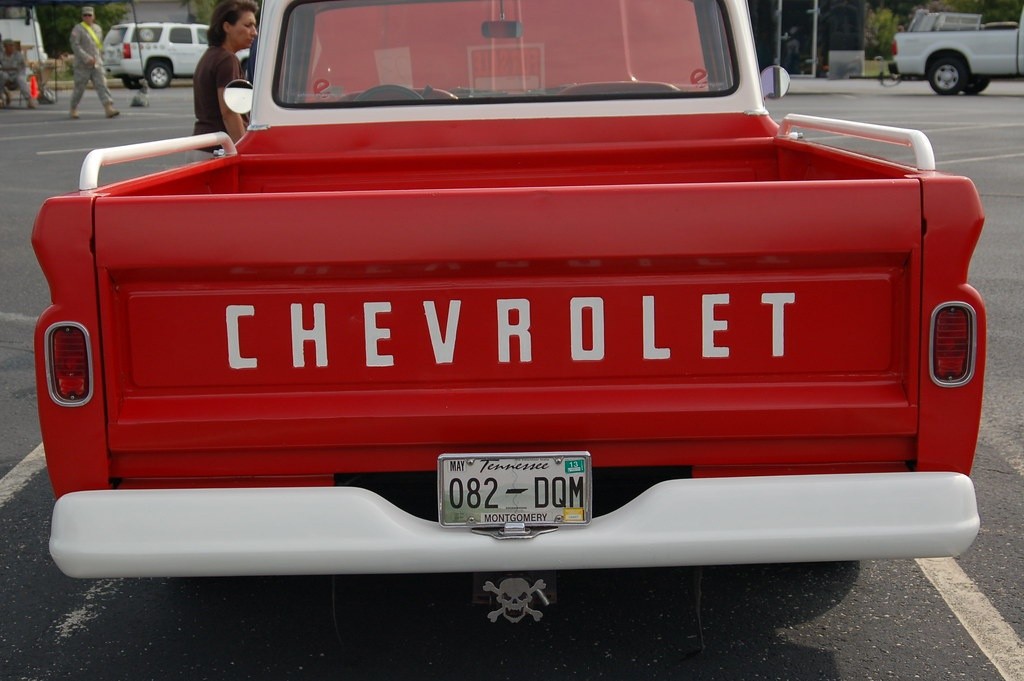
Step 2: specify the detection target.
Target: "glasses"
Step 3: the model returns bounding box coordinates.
[83,14,92,17]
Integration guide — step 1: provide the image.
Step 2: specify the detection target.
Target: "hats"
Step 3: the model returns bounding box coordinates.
[81,6,94,15]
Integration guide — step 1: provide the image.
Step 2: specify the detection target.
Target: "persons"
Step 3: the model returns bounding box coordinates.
[184,0,260,162]
[891,25,905,67]
[69,6,120,117]
[0,39,37,108]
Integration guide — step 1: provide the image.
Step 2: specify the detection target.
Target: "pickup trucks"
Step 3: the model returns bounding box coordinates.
[31,0,983,630]
[892,4,1024,95]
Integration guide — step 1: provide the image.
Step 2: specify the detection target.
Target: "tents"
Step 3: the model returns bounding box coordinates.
[1,0,145,111]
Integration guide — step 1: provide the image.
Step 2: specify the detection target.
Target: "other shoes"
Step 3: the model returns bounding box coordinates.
[68,107,79,118]
[105,105,119,117]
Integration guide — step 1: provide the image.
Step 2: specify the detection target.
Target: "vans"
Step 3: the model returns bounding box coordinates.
[101,22,252,89]
